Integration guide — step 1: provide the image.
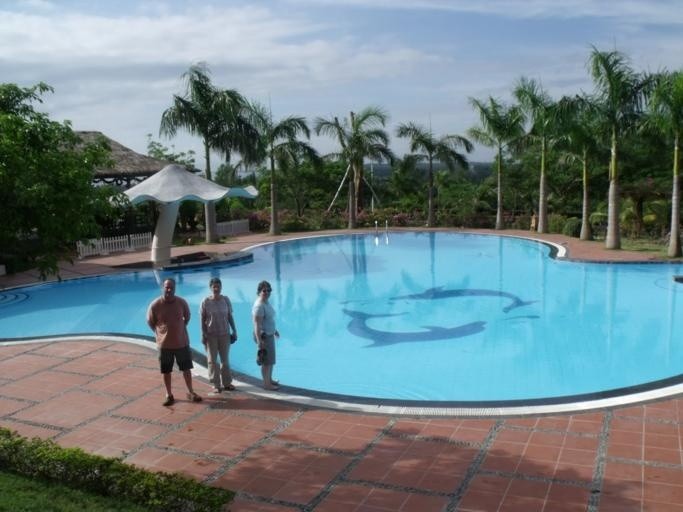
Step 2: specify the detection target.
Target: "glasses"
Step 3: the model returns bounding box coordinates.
[263,289,271,293]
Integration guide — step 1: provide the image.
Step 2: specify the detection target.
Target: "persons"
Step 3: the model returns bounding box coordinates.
[199,278,237,393]
[251,280,281,390]
[146,278,201,406]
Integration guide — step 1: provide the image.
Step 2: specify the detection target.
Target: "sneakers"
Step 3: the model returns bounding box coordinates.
[163,395,174,406]
[186,391,202,401]
